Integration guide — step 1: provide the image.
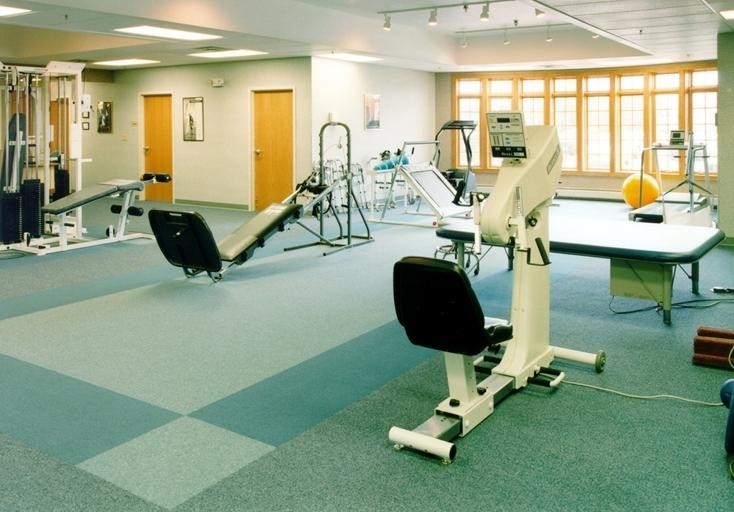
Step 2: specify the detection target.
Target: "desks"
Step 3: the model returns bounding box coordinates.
[436,218,725,325]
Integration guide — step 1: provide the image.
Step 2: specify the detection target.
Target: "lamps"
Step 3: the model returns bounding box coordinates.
[376,1,600,32]
[455,23,600,49]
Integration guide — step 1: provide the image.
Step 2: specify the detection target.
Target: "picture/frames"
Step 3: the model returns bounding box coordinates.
[82,101,112,133]
[364,94,381,129]
[181,96,204,141]
